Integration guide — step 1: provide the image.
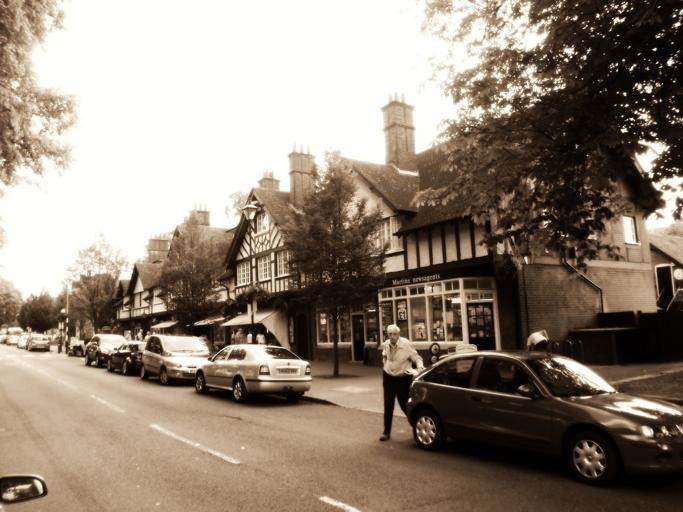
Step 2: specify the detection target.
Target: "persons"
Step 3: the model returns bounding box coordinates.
[125,331,131,341]
[137,329,144,341]
[145,331,150,342]
[379,324,424,447]
[526,328,550,352]
[491,361,524,394]
[147,342,154,350]
[231,328,267,344]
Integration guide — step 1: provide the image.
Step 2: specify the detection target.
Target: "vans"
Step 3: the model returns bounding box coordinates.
[85,333,127,369]
[140,334,211,384]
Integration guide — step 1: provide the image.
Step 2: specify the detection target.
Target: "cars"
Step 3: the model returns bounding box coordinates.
[406,350,683,491]
[0,323,50,351]
[106,340,147,376]
[195,343,312,402]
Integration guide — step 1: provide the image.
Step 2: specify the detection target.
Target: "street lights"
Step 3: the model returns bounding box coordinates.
[58,308,65,351]
[241,204,258,343]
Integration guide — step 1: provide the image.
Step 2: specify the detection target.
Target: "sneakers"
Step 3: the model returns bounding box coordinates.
[380,434,388,440]
